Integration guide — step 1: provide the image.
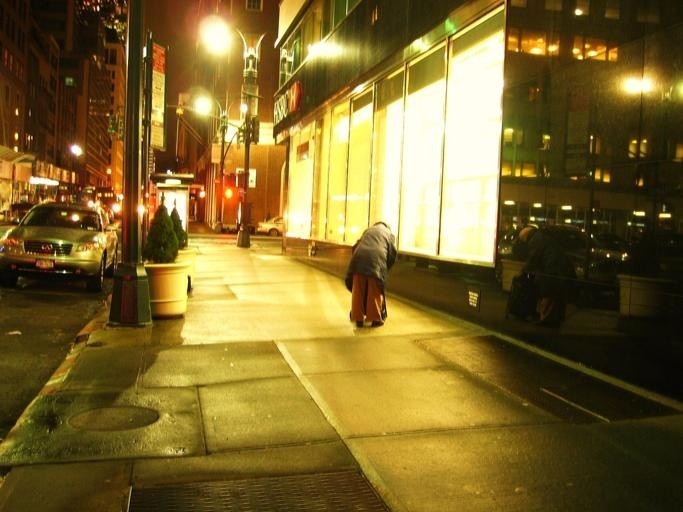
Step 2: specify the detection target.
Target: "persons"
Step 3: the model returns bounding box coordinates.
[510,221,574,327]
[347,221,395,328]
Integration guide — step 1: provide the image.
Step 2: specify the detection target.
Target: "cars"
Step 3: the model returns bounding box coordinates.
[0,200,119,294]
[255,215,283,237]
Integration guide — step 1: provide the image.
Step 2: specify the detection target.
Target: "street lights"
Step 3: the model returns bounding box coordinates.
[198,7,272,247]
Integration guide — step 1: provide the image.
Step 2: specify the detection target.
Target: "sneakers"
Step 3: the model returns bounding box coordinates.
[356,319,384,328]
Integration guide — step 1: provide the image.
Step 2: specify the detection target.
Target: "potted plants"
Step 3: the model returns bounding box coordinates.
[496,224,534,293]
[141,201,192,316]
[616,236,670,319]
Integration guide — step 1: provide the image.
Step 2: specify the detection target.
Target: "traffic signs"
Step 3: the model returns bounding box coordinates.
[190,88,229,234]
